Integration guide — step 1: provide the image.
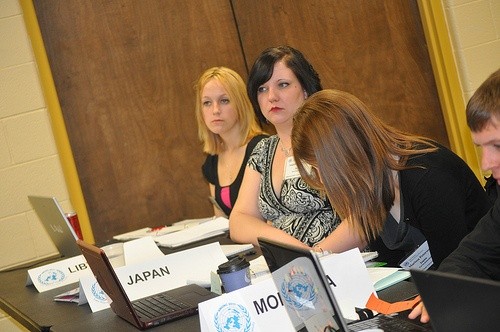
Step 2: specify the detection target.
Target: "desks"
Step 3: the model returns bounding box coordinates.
[0,222,264,332]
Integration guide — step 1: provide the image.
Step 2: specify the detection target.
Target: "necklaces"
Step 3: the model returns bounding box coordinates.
[279,139,294,157]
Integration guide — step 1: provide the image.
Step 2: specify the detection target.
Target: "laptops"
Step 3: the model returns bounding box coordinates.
[258,237,434,332]
[410,268,500,332]
[76,240,221,330]
[28,195,83,258]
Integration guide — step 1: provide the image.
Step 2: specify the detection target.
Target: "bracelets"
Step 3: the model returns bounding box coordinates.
[314,244,325,253]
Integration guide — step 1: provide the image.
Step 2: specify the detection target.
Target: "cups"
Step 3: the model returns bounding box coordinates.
[217,255,251,293]
[100,243,126,268]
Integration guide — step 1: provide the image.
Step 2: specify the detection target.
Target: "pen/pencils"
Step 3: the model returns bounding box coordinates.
[227,250,257,260]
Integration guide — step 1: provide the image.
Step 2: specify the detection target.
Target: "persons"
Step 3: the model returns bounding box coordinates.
[291,89,492,275]
[227,45,343,255]
[194,67,273,221]
[406,67,500,324]
